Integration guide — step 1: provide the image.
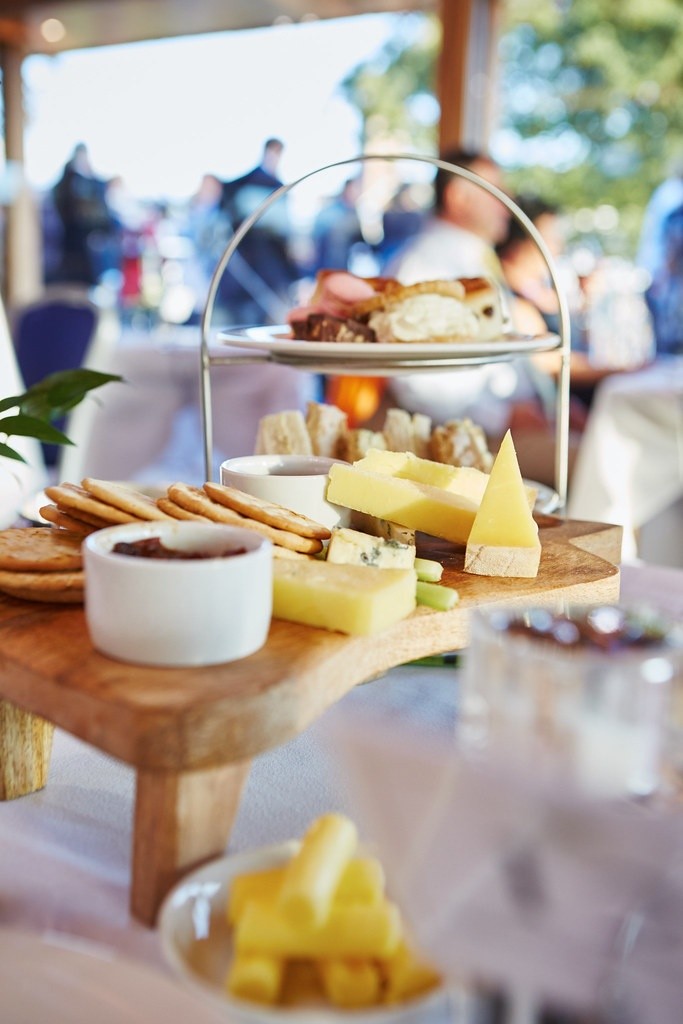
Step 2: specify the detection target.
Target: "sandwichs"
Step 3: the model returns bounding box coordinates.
[248,399,490,474]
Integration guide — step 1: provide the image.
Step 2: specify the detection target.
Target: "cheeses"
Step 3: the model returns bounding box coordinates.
[266,428,542,636]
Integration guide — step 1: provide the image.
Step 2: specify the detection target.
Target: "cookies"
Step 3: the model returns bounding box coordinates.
[1,475,329,607]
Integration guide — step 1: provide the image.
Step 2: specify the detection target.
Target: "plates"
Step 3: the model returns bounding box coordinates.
[157,843,445,1024]
[0,931,235,1024]
[18,494,57,528]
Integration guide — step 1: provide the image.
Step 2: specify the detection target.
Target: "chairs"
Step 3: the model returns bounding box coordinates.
[17,293,103,469]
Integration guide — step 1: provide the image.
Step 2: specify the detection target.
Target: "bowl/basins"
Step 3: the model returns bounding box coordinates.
[84,522,272,668]
[220,455,351,549]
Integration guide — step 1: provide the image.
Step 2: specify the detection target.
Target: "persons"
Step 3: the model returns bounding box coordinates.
[56,140,683,570]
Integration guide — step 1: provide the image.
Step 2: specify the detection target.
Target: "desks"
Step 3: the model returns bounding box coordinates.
[567,356,683,561]
[84,330,319,483]
[0,509,622,928]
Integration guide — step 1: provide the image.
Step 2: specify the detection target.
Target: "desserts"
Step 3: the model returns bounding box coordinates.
[283,266,506,343]
